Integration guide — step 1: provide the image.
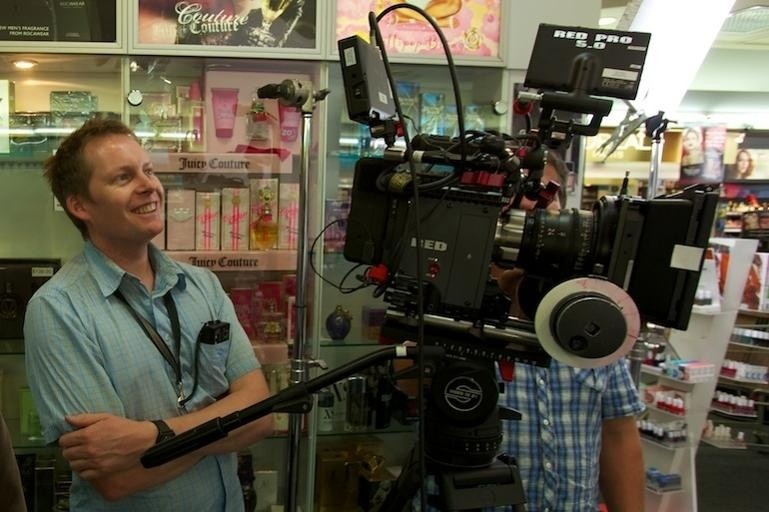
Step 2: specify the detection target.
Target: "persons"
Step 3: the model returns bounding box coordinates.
[22,117,277,512]
[482,149,651,512]
[733,150,753,178]
[682,128,704,166]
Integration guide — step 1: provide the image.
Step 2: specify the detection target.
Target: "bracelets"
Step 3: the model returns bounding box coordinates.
[148,418,178,446]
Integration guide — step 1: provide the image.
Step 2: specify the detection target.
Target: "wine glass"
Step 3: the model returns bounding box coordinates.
[255,0,292,38]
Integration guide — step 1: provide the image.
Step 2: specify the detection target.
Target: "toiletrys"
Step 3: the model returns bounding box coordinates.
[251,182,282,253]
[319,369,395,432]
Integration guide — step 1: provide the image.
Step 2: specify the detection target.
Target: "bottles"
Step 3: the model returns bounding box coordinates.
[324,303,353,341]
[246,90,267,142]
[703,329,769,452]
[250,186,280,253]
[635,391,688,444]
[344,375,394,431]
[317,391,334,432]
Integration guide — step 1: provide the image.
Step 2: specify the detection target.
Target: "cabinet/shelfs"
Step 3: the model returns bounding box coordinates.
[0,47,514,512]
[642,236,769,512]
